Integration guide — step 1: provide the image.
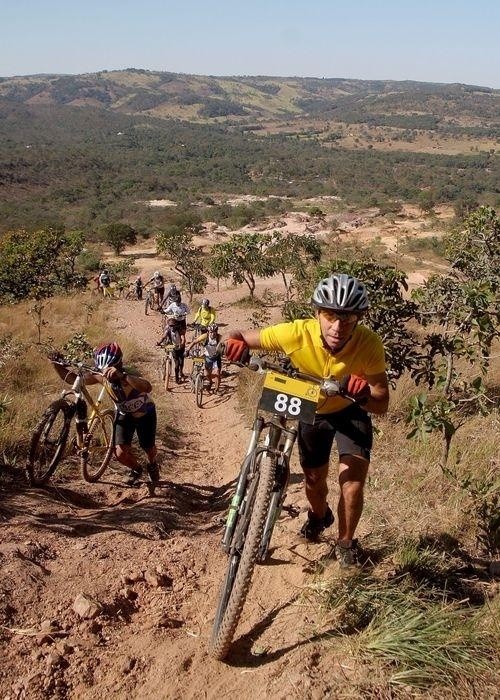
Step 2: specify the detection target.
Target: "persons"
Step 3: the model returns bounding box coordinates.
[221,274,389,577]
[93,269,223,396]
[48,342,160,484]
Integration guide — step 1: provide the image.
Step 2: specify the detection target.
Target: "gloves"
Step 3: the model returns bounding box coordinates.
[340,374,371,400]
[48,352,65,363]
[224,338,251,367]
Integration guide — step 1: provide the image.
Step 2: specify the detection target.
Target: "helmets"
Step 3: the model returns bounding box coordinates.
[201,298,209,306]
[167,318,177,326]
[174,295,181,302]
[170,284,176,295]
[94,342,123,371]
[312,272,369,313]
[154,272,160,279]
[208,324,218,332]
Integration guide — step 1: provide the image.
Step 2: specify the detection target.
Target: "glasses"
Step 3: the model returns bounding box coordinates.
[319,309,358,325]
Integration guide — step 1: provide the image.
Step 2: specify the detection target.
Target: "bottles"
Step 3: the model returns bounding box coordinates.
[78,399,89,434]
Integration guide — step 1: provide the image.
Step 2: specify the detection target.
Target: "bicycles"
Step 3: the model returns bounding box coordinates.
[208,341,371,663]
[23,351,117,484]
[116,281,142,300]
[155,298,214,408]
[143,285,158,316]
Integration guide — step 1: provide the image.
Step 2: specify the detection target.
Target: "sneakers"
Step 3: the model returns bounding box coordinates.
[214,390,222,396]
[207,380,212,391]
[127,465,143,485]
[335,538,366,574]
[180,374,188,379]
[147,458,159,482]
[301,502,334,539]
[176,379,182,384]
